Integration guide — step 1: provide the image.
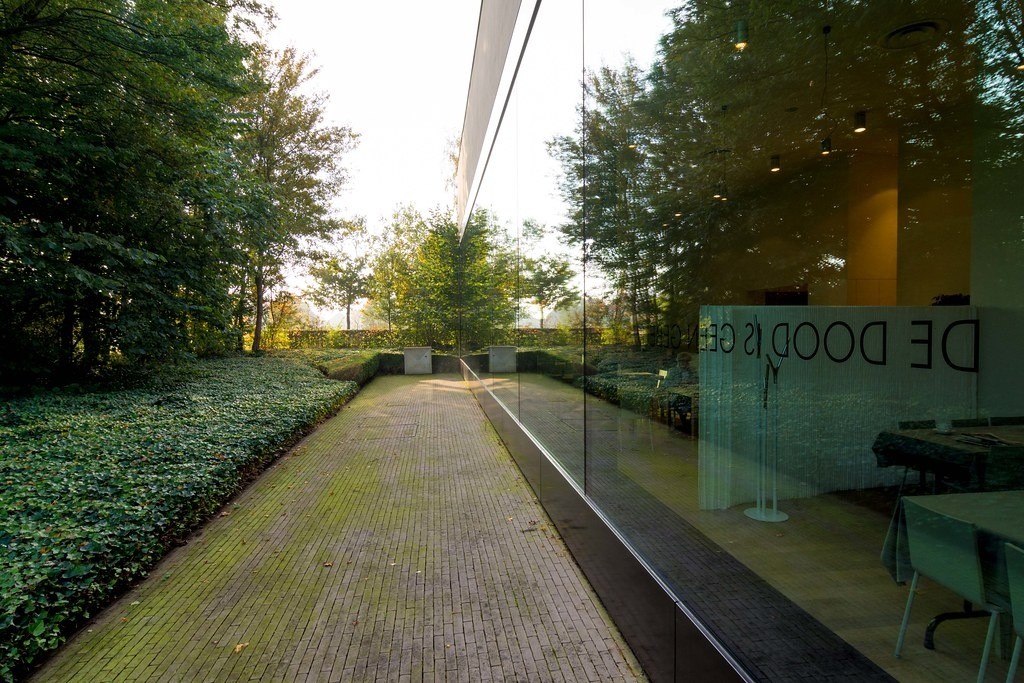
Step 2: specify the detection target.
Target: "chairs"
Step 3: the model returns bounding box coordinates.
[887,417,1024,683]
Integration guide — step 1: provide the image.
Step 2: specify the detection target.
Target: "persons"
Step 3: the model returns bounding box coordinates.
[661,353,699,434]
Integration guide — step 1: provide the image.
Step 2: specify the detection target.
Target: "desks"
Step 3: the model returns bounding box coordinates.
[872,425,1024,514]
[876,489,1024,585]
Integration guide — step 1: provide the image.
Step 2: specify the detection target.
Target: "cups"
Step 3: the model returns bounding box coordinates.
[935,414,952,431]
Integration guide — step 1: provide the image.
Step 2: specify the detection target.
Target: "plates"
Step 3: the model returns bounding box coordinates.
[932,428,957,435]
